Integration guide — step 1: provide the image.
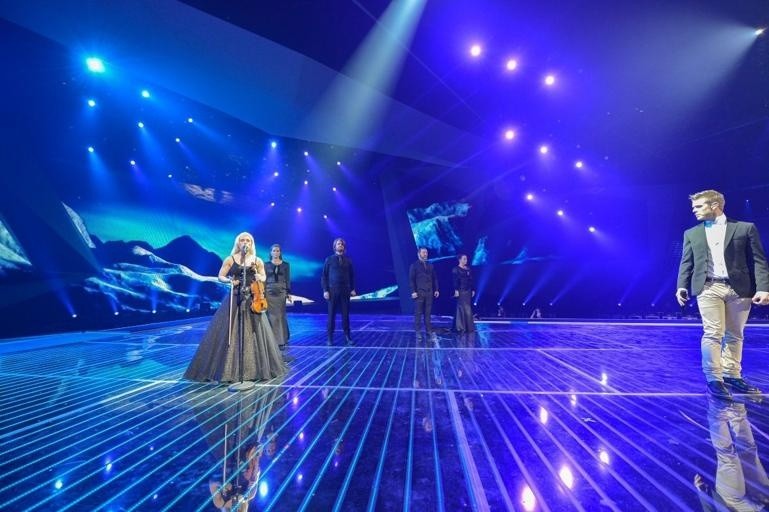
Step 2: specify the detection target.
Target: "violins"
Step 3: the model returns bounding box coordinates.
[250,262,268,313]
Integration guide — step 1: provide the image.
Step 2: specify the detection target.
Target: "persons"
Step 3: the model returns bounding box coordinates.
[182,231,285,383]
[675,187,769,401]
[182,388,277,512]
[694,391,769,512]
[262,243,293,351]
[450,253,477,333]
[454,335,484,413]
[409,248,440,338]
[414,356,448,437]
[320,237,357,346]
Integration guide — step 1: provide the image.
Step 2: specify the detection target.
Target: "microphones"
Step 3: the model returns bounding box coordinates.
[243,245,248,254]
[680,290,686,310]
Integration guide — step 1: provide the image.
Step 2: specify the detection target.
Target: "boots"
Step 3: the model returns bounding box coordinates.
[327,334,333,346]
[345,334,354,345]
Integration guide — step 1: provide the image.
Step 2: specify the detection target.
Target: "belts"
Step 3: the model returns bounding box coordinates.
[705,276,730,284]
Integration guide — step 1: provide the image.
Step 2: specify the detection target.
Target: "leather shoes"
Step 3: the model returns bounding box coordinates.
[415,330,423,338]
[706,376,756,399]
[426,329,432,337]
